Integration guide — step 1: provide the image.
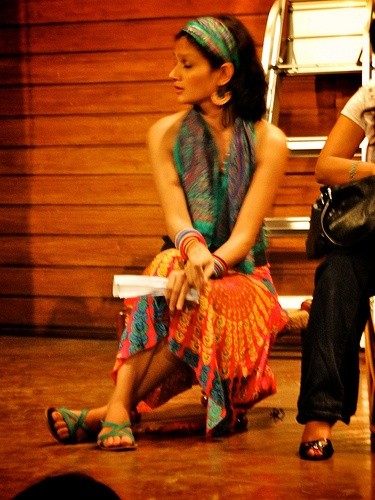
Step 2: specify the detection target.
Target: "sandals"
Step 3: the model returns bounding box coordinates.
[300,439,334,461]
[46,405,99,444]
[98,422,138,450]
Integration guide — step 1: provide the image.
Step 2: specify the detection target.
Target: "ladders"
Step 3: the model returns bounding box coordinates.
[258,1,374,421]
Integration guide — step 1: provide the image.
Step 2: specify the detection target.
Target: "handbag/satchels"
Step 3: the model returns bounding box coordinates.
[306,175,375,259]
